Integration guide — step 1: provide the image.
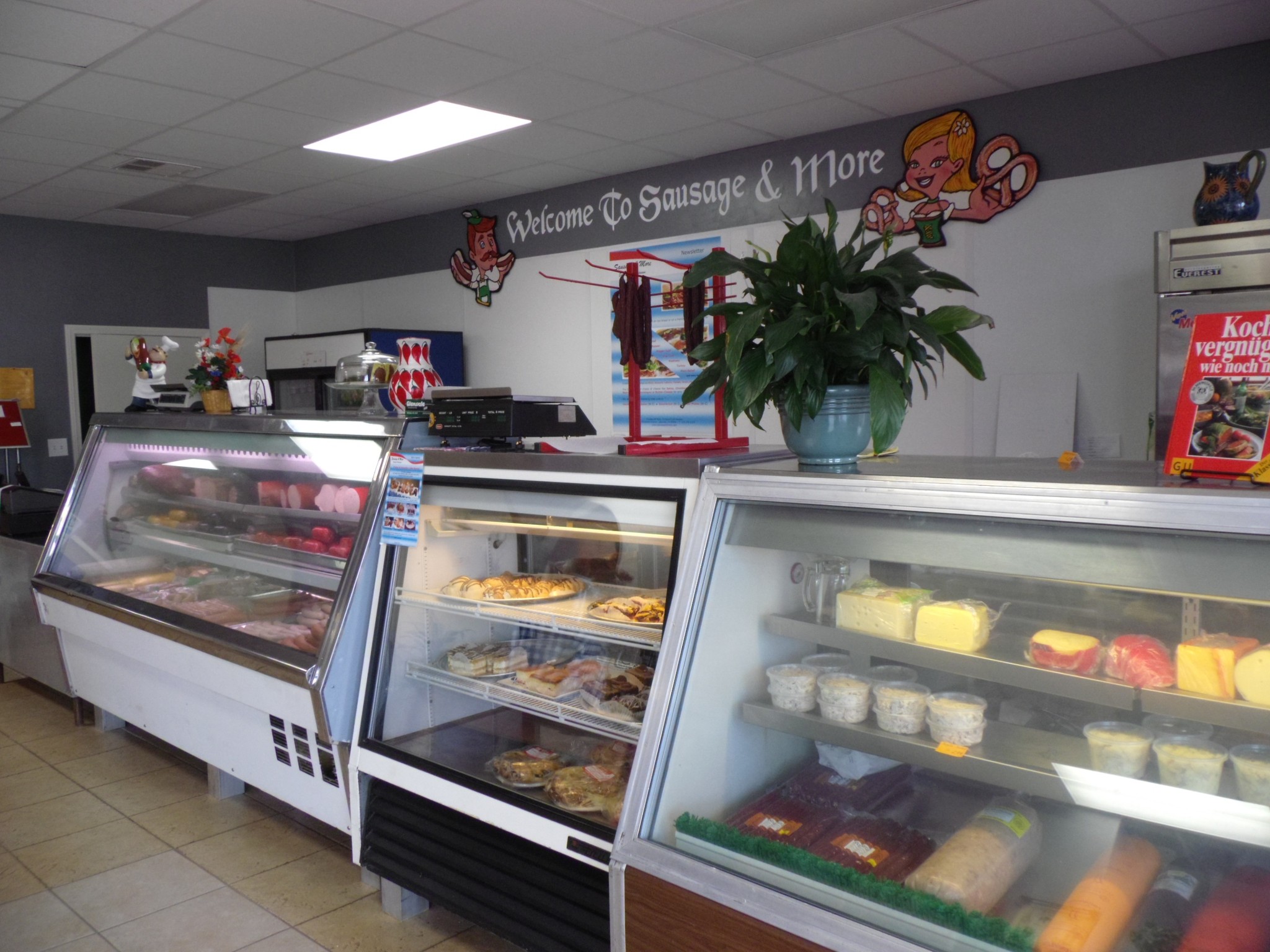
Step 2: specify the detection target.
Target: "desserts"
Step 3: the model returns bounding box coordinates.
[591,595,665,623]
[442,573,585,601]
[446,642,657,719]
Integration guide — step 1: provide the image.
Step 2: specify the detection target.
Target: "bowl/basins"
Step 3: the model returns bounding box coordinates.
[1247,384,1270,405]
[764,664,820,711]
[800,653,919,684]
[870,682,930,734]
[816,674,873,724]
[1194,410,1213,427]
[927,691,988,746]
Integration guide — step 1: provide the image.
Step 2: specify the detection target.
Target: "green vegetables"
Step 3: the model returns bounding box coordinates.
[1229,404,1269,428]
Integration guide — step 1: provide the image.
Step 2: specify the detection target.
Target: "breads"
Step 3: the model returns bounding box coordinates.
[490,739,636,824]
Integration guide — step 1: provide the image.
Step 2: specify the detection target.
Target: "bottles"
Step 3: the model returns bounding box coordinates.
[1035,833,1160,952]
[904,789,1042,917]
[1234,377,1248,416]
[388,337,444,414]
[1130,847,1214,952]
[1176,861,1270,952]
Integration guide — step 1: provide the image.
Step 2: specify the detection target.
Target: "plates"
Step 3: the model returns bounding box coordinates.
[437,574,665,628]
[1227,414,1266,431]
[1193,427,1264,461]
[493,771,601,812]
[497,666,644,725]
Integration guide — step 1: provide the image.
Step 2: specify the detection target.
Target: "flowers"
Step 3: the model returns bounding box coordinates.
[184,326,246,396]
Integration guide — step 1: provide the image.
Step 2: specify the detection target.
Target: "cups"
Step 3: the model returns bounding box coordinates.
[1230,743,1270,807]
[1152,736,1230,795]
[801,561,849,627]
[1082,720,1154,779]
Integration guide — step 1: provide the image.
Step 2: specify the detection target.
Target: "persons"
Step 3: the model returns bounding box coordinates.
[124,335,179,412]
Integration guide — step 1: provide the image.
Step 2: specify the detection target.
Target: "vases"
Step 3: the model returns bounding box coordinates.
[200,390,232,415]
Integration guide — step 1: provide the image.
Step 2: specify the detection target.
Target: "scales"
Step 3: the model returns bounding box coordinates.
[150,382,204,413]
[427,386,597,450]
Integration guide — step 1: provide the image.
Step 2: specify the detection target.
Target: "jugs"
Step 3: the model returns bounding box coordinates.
[1192,149,1266,227]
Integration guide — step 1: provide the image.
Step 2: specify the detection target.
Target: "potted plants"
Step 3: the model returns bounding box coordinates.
[675,197,993,465]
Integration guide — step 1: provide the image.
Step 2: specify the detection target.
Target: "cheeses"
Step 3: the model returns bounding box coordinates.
[833,584,1270,705]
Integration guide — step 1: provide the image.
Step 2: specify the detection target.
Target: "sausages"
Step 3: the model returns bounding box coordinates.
[95,563,333,655]
[725,758,938,885]
[143,510,356,558]
